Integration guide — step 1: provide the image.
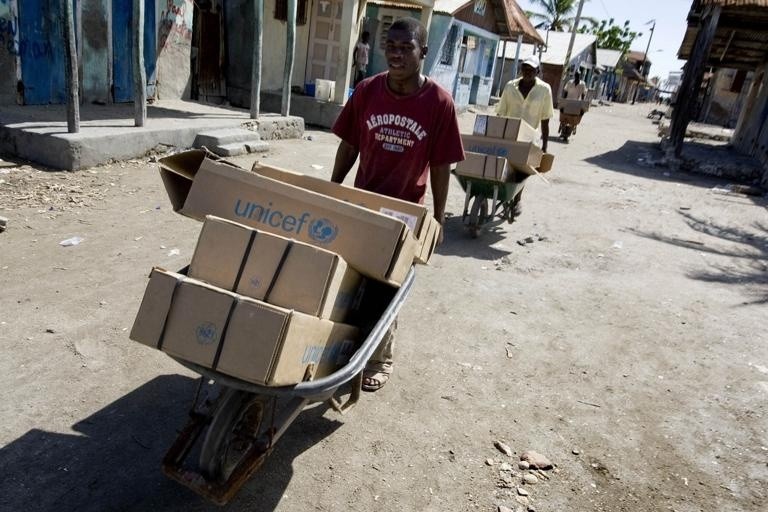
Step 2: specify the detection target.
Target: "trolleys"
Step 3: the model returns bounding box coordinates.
[153,254,420,502]
[558,111,583,143]
[448,168,540,237]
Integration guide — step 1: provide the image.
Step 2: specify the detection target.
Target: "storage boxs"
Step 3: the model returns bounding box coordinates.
[557,98,590,116]
[130,265,363,388]
[186,216,362,326]
[454,113,554,184]
[178,157,443,288]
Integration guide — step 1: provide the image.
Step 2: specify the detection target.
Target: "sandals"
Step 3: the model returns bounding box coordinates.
[362,370,390,390]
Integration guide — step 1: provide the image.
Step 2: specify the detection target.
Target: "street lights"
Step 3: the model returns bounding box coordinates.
[632,19,656,105]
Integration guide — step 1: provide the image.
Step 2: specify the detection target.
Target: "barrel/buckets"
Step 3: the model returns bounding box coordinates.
[330,81,336,102]
[306,83,315,96]
[314,78,330,103]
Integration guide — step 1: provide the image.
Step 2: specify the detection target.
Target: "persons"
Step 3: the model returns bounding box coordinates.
[327,14,462,392]
[561,70,587,102]
[497,55,554,218]
[351,31,371,83]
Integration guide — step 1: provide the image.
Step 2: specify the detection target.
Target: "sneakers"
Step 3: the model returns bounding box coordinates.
[514,202,521,214]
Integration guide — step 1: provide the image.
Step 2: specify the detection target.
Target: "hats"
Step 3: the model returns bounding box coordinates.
[521,56,539,68]
